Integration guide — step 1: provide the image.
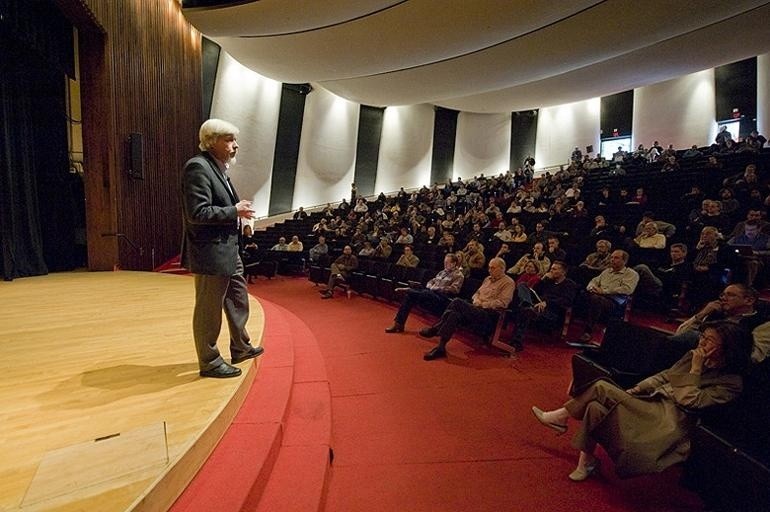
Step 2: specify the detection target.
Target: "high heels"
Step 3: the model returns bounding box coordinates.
[532,406,569,437]
[569,457,600,482]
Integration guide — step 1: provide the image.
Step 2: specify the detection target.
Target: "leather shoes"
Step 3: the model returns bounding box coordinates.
[231,346,264,365]
[418,323,443,339]
[423,347,447,361]
[199,361,242,379]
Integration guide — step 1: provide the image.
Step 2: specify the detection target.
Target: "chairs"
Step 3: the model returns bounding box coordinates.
[569,147,769,511]
[308,198,393,302]
[253,219,308,280]
[393,174,569,358]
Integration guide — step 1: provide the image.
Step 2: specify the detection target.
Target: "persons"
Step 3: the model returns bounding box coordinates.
[179,119,264,379]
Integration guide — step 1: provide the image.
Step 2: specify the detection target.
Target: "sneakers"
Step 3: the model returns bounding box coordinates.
[385,323,405,333]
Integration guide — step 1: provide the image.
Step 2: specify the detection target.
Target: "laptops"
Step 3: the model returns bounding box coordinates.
[728,244,753,256]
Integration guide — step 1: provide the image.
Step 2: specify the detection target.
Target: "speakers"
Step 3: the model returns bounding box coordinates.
[131,132,145,179]
